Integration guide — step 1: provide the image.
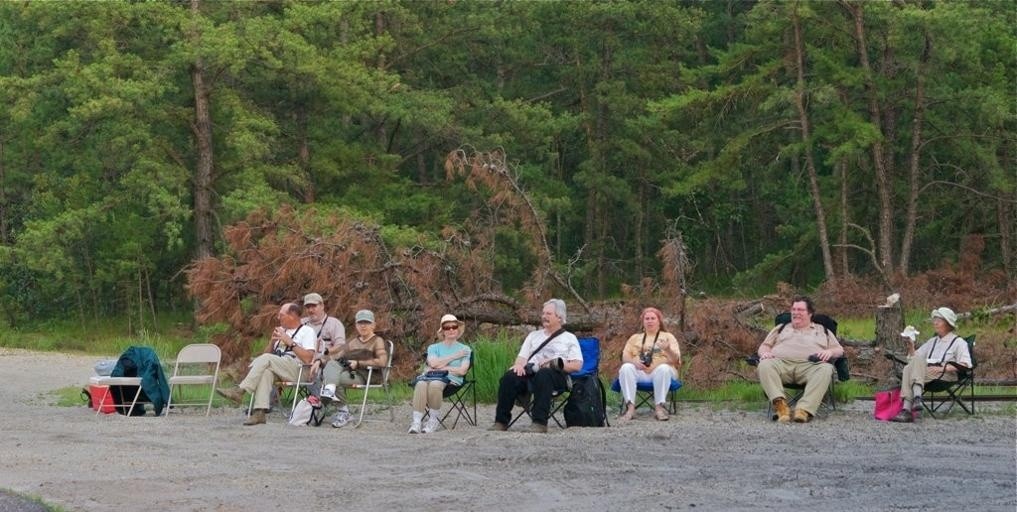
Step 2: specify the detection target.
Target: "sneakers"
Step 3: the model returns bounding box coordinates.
[892,407,915,423]
[654,407,672,422]
[770,396,792,427]
[215,385,246,405]
[914,401,926,416]
[619,409,637,424]
[320,388,342,403]
[486,421,507,433]
[520,422,547,436]
[406,418,441,434]
[792,406,813,425]
[330,408,354,428]
[242,409,268,427]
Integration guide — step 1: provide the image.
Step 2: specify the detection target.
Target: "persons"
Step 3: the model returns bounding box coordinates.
[756,296,844,423]
[407,313,472,433]
[616,307,682,420]
[889,307,973,422]
[486,298,585,432]
[215,292,388,428]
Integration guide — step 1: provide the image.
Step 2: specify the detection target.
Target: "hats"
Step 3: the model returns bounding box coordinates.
[929,305,960,331]
[354,309,377,324]
[435,313,466,341]
[302,292,324,307]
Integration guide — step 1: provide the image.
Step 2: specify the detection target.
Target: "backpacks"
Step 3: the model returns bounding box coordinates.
[562,372,610,428]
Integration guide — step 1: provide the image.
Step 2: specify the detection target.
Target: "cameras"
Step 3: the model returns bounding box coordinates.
[640,351,653,366]
[273,350,281,357]
[523,356,564,378]
[808,353,833,363]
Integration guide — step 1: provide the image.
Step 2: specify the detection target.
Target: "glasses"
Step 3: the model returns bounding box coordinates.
[442,325,459,331]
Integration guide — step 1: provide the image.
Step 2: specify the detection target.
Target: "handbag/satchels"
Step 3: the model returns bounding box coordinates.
[873,384,919,422]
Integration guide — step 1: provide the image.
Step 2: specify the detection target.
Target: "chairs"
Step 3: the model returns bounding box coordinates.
[559,336,611,428]
[247,334,329,421]
[405,342,478,433]
[882,331,979,420]
[614,350,680,418]
[94,346,159,415]
[765,314,845,415]
[167,341,225,418]
[506,368,571,431]
[316,337,399,427]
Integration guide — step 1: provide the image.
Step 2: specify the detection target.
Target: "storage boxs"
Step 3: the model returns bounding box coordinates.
[88,376,117,414]
